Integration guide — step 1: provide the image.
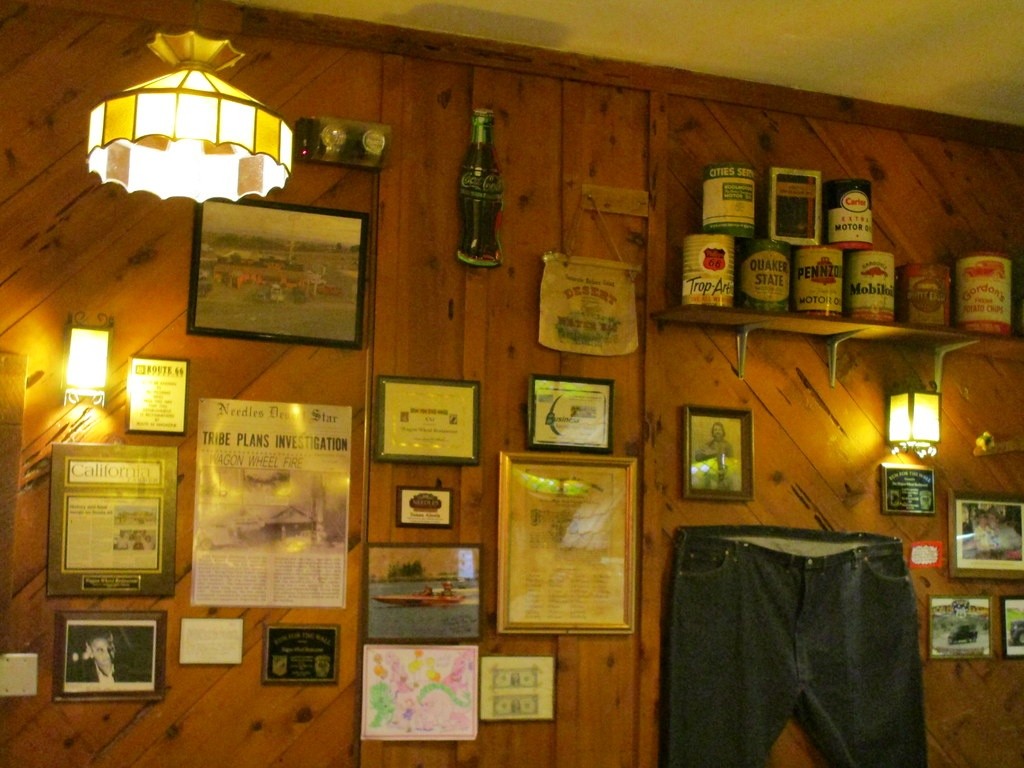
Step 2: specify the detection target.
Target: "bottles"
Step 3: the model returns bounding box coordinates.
[457,107,504,268]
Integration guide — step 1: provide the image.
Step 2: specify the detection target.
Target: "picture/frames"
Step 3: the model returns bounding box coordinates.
[683,404,755,501]
[928,594,994,659]
[53,609,168,703]
[373,374,481,467]
[1001,595,1024,659]
[123,355,187,436]
[359,646,478,743]
[882,463,935,515]
[526,373,616,453]
[480,652,556,721]
[189,204,368,348]
[361,543,487,642]
[496,449,637,634]
[396,484,455,529]
[46,442,178,594]
[948,487,1024,580]
[262,621,341,686]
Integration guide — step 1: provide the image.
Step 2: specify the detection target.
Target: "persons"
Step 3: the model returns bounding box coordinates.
[66,628,142,684]
[973,507,1021,561]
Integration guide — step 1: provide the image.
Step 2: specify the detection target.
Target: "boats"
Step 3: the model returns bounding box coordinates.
[373,594,465,608]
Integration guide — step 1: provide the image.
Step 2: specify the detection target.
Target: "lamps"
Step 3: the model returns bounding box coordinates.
[888,379,940,457]
[297,114,392,168]
[61,310,118,406]
[87,30,296,205]
